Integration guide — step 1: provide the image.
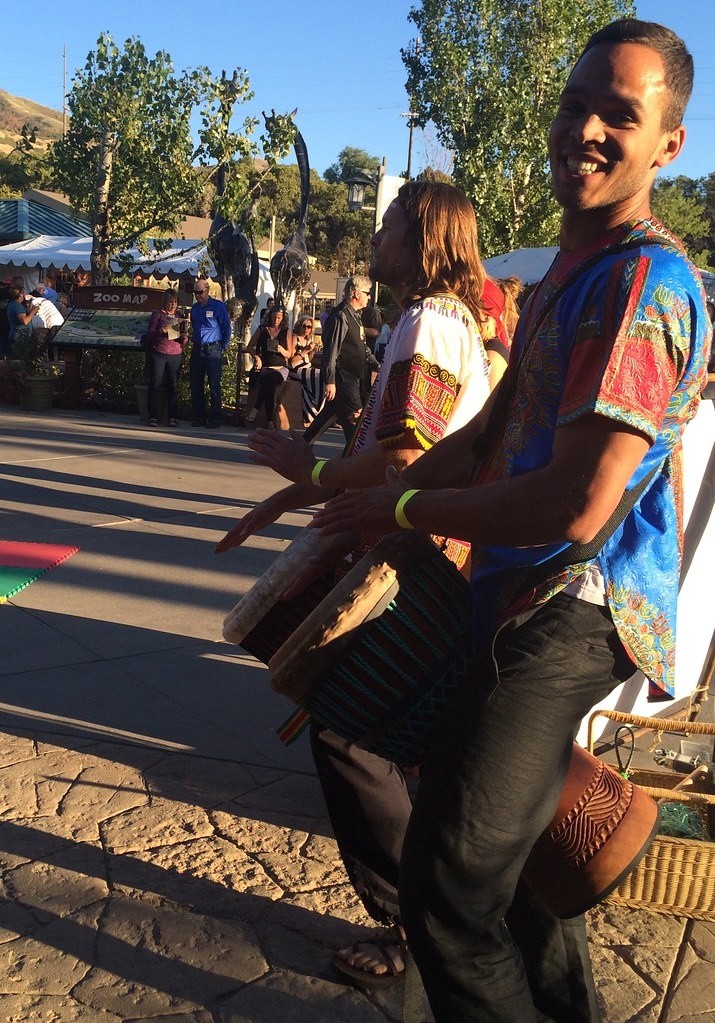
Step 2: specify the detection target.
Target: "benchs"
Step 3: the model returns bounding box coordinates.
[246,378,305,430]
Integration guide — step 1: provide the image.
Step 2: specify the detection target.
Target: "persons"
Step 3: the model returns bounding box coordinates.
[0,270,89,373]
[246,298,343,430]
[305,275,393,447]
[189,280,231,428]
[480,276,521,384]
[147,288,189,427]
[313,18,715,1022]
[217,183,494,985]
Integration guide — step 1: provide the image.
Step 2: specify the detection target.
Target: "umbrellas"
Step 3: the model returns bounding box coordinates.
[483,248,559,286]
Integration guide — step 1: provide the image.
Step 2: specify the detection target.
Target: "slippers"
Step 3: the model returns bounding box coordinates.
[245,415,256,423]
[267,427,275,432]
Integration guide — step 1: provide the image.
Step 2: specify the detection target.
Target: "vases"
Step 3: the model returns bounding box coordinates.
[134,385,170,425]
[17,378,57,411]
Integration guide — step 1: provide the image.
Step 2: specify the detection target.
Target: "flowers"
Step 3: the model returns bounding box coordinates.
[8,327,67,379]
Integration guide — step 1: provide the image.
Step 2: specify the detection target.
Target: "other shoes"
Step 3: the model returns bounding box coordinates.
[192,419,207,426]
[328,422,344,430]
[208,420,221,428]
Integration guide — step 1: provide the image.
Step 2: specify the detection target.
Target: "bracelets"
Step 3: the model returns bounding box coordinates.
[394,490,419,529]
[252,353,257,356]
[312,460,327,487]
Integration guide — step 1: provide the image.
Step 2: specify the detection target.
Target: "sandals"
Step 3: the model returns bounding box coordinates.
[330,924,408,989]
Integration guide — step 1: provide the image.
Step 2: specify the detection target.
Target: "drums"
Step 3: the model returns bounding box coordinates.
[265,529,666,927]
[217,508,388,669]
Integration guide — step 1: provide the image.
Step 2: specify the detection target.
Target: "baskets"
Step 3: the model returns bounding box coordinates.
[587,710,715,922]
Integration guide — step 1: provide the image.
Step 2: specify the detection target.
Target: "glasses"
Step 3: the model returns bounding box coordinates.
[193,288,208,295]
[361,291,370,296]
[21,287,25,291]
[303,325,312,329]
[325,306,332,308]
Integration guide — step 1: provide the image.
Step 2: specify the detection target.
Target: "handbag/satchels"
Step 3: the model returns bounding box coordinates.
[25,308,44,331]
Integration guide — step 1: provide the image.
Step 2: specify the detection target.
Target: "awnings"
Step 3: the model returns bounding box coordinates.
[0,234,216,304]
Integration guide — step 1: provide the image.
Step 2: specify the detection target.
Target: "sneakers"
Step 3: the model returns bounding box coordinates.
[149,417,158,426]
[168,418,176,426]
